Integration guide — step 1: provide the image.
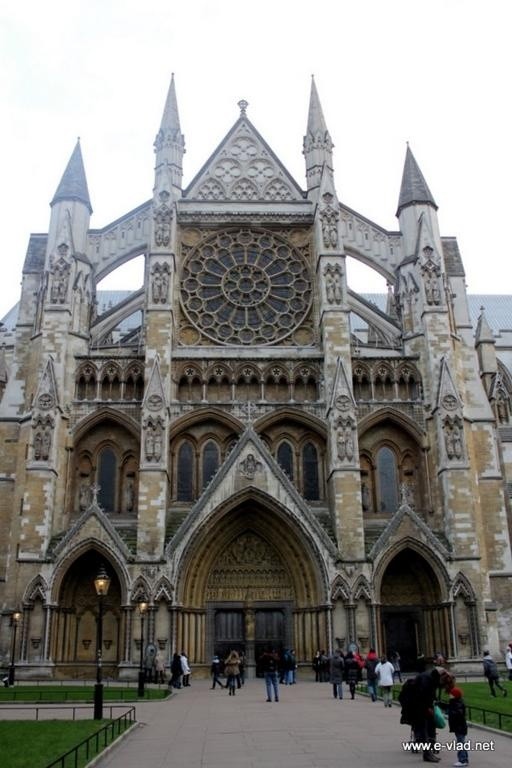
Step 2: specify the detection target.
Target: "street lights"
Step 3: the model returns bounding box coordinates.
[8,605,23,688]
[133,593,150,697]
[93,564,112,721]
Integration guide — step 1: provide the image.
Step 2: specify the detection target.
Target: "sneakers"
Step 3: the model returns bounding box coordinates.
[453,762,468,766]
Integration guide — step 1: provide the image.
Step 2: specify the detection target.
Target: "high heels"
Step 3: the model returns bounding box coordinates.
[423,751,441,762]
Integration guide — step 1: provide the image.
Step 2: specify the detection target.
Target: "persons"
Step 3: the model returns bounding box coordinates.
[142,647,447,707]
[434,687,469,767]
[481,648,507,700]
[505,642,511,684]
[398,668,455,766]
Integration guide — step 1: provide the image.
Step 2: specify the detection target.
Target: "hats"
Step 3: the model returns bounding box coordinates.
[450,688,462,698]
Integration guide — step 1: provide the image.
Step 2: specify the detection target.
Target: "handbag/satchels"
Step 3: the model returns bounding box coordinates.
[432,704,445,728]
